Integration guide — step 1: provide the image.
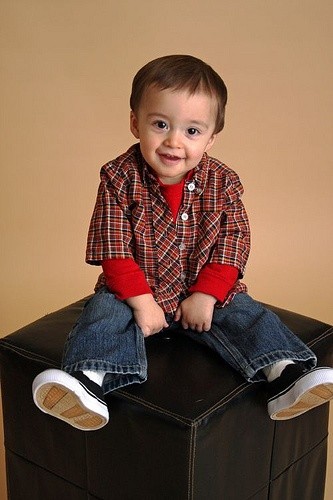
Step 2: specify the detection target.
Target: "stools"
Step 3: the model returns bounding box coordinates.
[0,293,333,499]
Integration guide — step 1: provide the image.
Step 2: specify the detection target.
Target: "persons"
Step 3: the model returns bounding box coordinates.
[31,54,333,432]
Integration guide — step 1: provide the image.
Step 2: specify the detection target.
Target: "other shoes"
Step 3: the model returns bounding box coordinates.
[31,368,109,430]
[267,363,333,420]
